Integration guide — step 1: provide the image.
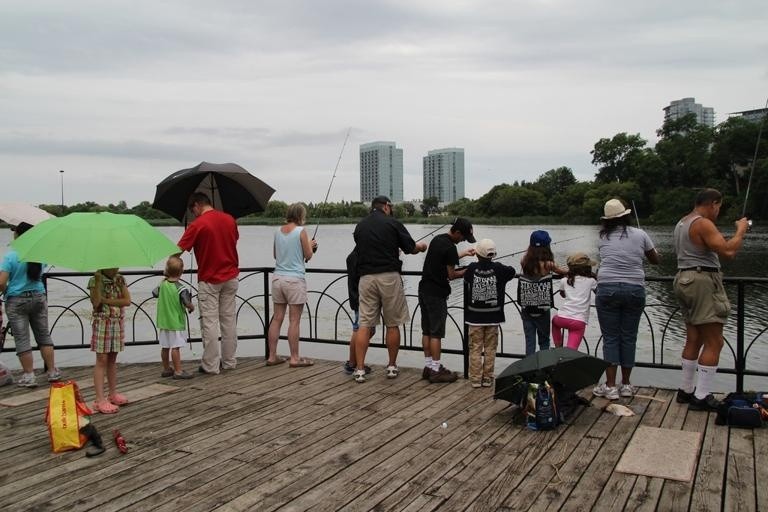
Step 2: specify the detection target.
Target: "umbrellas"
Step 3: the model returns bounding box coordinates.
[9,211,186,277]
[148,161,275,224]
[492,344,613,424]
[0,201,60,229]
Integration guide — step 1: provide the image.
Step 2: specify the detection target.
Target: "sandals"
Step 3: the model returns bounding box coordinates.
[93,399,119,414]
[173,370,194,380]
[161,367,174,377]
[107,393,128,405]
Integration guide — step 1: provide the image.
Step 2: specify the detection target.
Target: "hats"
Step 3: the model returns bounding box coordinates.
[454,217,476,243]
[475,239,497,258]
[567,252,597,267]
[372,196,394,217]
[600,199,632,220]
[530,230,551,246]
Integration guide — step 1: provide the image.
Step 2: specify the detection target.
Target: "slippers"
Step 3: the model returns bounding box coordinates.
[290,357,314,367]
[266,357,286,366]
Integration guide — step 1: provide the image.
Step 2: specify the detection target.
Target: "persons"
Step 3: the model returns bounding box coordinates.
[87,267,133,416]
[344,243,377,375]
[351,193,428,385]
[263,202,319,368]
[170,191,241,373]
[417,217,477,384]
[0,221,63,387]
[461,238,517,389]
[672,186,749,414]
[551,252,597,352]
[152,255,197,380]
[516,228,570,360]
[588,195,663,402]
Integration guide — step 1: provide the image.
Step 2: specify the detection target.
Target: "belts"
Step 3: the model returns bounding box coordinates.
[681,265,720,273]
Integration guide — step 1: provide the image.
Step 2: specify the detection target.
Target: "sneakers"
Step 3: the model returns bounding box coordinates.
[344,360,372,383]
[676,386,697,404]
[688,393,721,412]
[386,365,401,379]
[18,375,39,387]
[422,364,458,383]
[483,378,492,387]
[472,380,481,388]
[47,368,62,381]
[593,381,620,400]
[621,384,634,397]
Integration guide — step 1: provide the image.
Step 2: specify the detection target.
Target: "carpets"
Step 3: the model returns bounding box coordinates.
[85,382,181,414]
[614,424,703,484]
[0,375,109,407]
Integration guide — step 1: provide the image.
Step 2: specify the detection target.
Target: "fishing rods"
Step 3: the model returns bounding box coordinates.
[305,126,349,262]
[742,100,768,218]
[413,224,445,243]
[455,236,583,270]
[631,198,640,230]
[189,248,193,313]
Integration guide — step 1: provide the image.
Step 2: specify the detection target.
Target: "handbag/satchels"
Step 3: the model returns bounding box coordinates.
[525,381,560,432]
[45,381,92,453]
[727,390,763,428]
